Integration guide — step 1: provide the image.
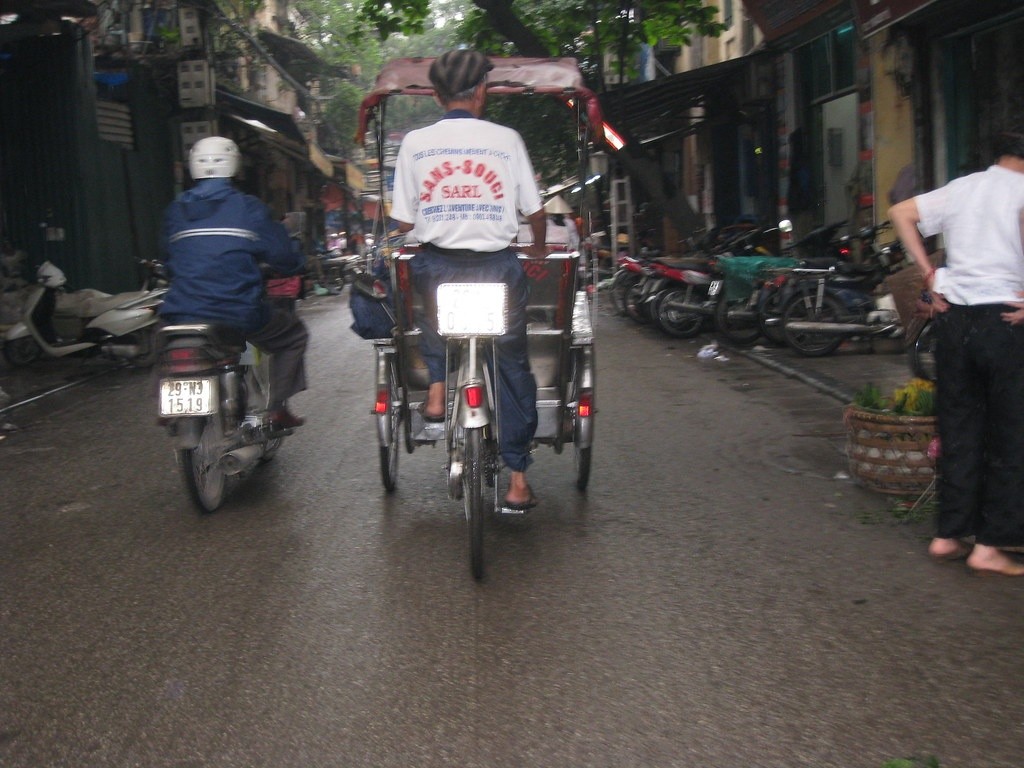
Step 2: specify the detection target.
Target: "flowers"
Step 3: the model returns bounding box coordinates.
[851,380,942,417]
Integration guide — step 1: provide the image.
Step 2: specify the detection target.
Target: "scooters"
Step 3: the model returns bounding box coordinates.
[0,255,172,374]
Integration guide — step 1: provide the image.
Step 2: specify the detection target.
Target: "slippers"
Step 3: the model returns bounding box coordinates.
[417,403,446,423]
[507,482,537,509]
[934,540,976,564]
[970,567,1024,579]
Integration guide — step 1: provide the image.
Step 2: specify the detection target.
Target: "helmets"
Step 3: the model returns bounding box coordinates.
[189,136,243,181]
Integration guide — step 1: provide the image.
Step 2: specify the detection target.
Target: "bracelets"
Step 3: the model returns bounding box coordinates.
[924,268,934,286]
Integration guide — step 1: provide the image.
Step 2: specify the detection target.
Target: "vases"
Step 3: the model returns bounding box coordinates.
[843,404,938,497]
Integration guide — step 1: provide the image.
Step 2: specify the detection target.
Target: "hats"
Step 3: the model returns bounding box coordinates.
[428,50,494,96]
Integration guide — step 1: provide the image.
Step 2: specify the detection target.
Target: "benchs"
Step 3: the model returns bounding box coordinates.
[395,238,578,389]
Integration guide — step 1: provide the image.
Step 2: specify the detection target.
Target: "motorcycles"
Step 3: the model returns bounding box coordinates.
[316,228,365,296]
[154,231,300,518]
[611,217,941,355]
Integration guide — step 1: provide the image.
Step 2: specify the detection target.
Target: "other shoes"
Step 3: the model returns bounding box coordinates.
[268,409,305,431]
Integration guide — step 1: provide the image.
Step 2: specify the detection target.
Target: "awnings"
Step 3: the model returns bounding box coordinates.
[576,50,769,157]
[260,28,348,82]
[542,173,604,198]
[217,82,368,191]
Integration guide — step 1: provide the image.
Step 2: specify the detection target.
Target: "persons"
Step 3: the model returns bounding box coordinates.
[889,131,1024,576]
[158,136,309,425]
[386,49,546,508]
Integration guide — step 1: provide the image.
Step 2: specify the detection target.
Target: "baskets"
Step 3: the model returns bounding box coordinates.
[845,408,943,495]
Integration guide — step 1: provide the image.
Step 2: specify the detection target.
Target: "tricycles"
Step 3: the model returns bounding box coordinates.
[348,48,605,588]
